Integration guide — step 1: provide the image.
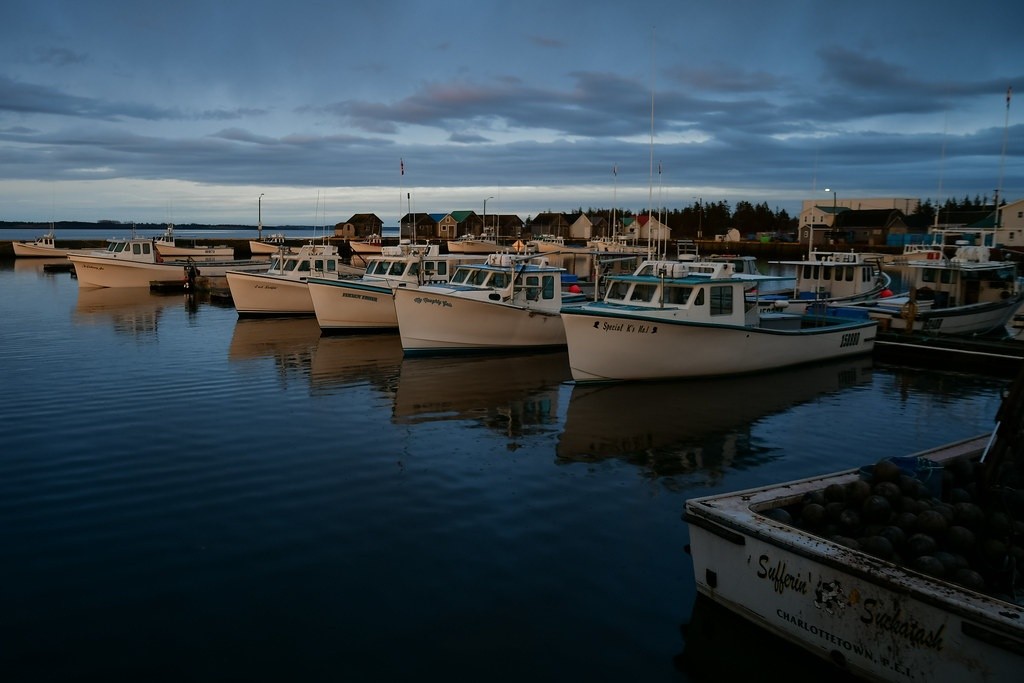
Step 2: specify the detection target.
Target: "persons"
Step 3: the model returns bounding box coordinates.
[364,232,380,243]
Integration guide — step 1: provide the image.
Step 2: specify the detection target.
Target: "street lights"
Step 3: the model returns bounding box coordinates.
[483,196,494,233]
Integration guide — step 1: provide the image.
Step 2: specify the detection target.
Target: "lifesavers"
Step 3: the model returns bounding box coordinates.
[900,301,918,319]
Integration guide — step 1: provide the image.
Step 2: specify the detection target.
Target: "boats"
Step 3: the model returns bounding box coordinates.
[388,351,570,426]
[309,332,402,392]
[224,241,366,317]
[305,253,480,336]
[1004,297,1024,342]
[11,220,106,258]
[154,207,235,256]
[391,252,612,358]
[227,315,320,364]
[69,287,188,323]
[64,221,272,289]
[857,86,1024,335]
[379,75,700,265]
[550,354,878,466]
[559,258,878,386]
